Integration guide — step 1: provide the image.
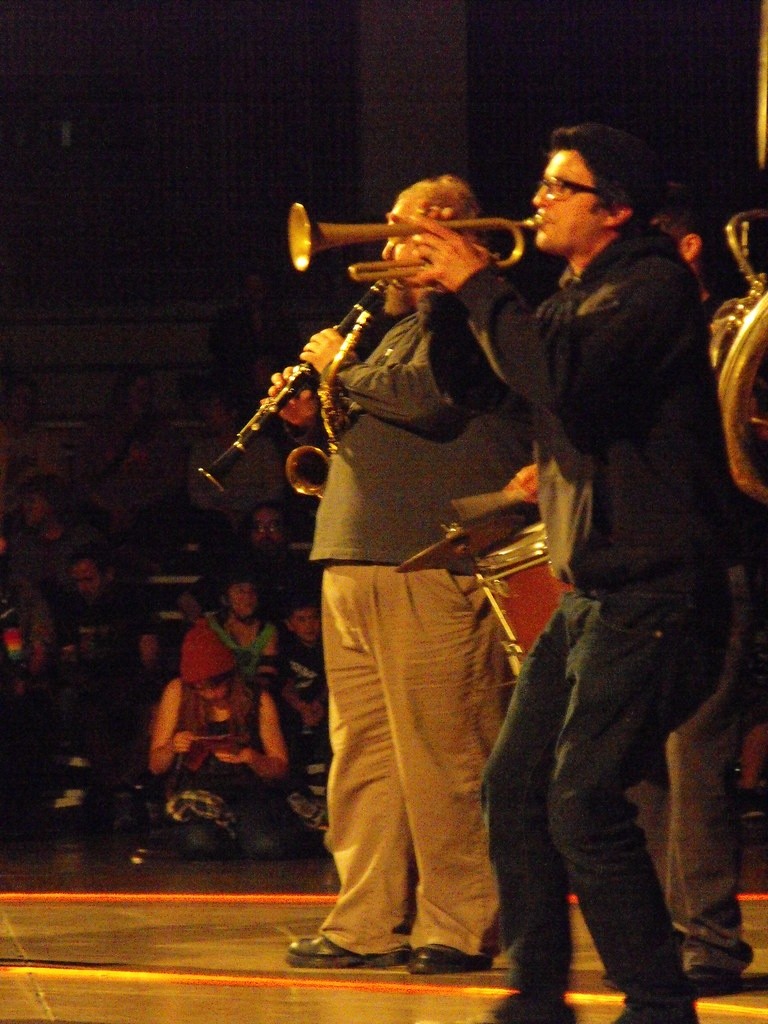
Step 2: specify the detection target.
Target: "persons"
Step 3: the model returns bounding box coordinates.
[0,270,333,860]
[260,124,767,1024]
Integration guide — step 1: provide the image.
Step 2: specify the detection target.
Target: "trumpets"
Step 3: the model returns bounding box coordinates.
[282,437,328,497]
[287,198,542,283]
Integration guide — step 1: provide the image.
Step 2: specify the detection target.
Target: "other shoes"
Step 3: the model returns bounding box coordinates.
[408,944,493,975]
[285,936,397,968]
[680,966,742,996]
[602,972,618,989]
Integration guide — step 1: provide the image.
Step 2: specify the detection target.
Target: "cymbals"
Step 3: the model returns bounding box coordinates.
[396,512,518,573]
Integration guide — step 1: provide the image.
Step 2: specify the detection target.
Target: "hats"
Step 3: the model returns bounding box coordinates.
[180,617,237,682]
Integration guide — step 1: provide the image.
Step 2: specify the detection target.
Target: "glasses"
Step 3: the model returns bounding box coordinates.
[536,176,601,200]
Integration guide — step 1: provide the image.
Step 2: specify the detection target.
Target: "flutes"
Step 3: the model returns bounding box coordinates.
[197,279,387,492]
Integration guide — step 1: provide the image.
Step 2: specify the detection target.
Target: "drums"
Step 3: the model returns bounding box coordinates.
[471,517,571,681]
